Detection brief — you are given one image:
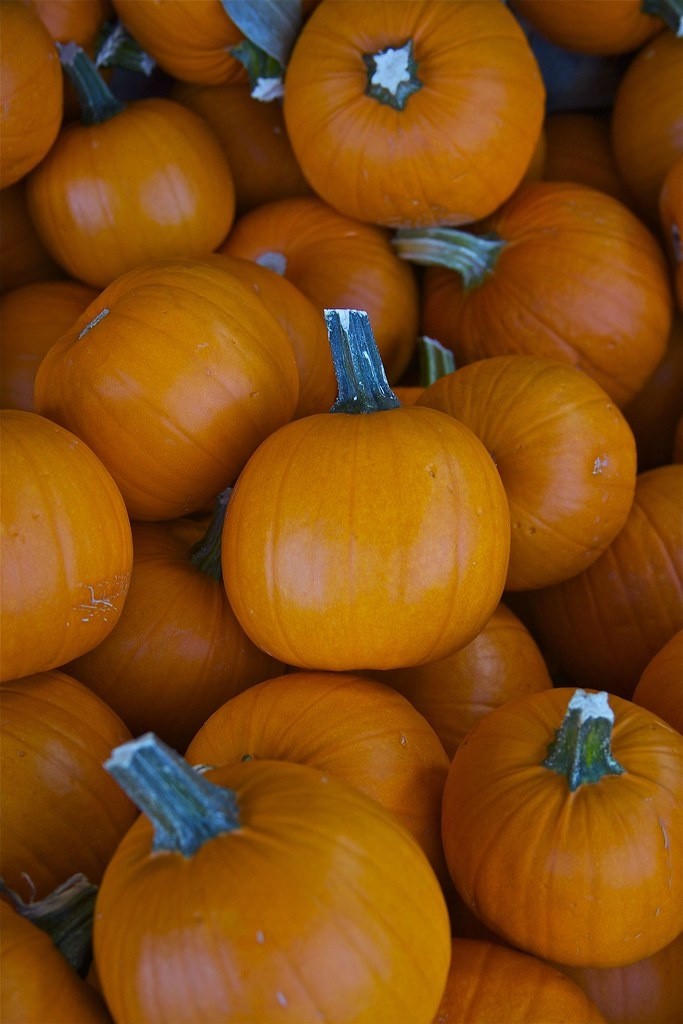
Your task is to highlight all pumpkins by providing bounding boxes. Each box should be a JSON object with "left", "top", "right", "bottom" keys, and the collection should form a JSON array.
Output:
[{"left": 2, "top": 0, "right": 683, "bottom": 1023}]
